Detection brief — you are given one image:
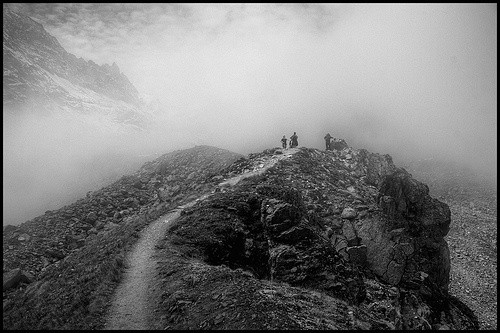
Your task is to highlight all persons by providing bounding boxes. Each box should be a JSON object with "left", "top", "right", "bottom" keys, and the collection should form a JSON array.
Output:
[
  {"left": 291, "top": 132, "right": 298, "bottom": 146},
  {"left": 323, "top": 133, "right": 333, "bottom": 148},
  {"left": 280, "top": 136, "right": 287, "bottom": 147}
]
